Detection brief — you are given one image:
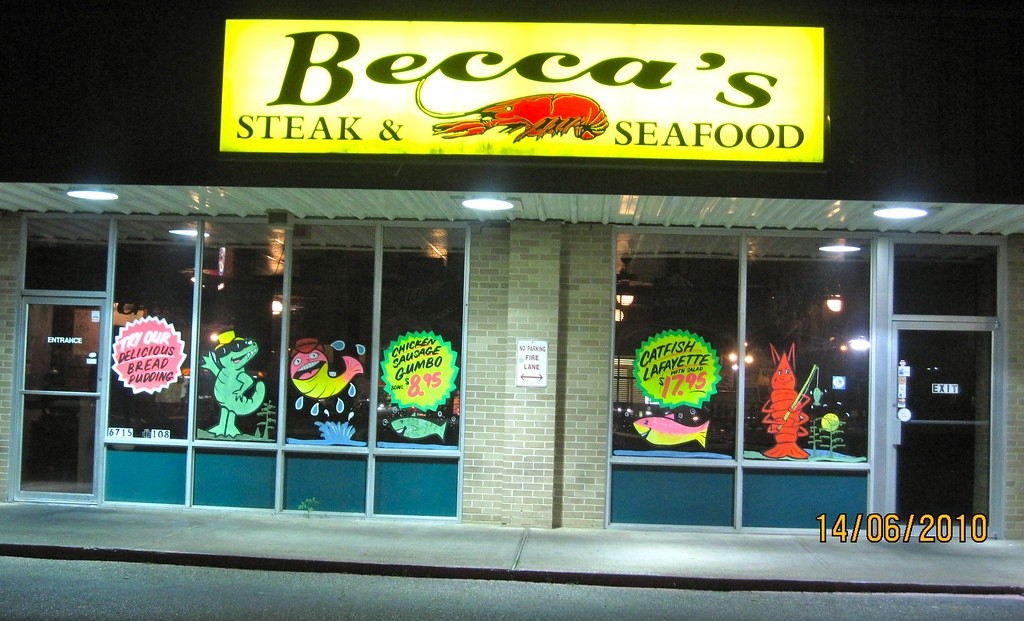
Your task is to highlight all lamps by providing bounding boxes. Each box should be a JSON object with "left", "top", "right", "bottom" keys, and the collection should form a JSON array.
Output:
[
  {"left": 825, "top": 286, "right": 843, "bottom": 312},
  {"left": 615, "top": 309, "right": 624, "bottom": 323},
  {"left": 616, "top": 281, "right": 634, "bottom": 306},
  {"left": 270, "top": 300, "right": 283, "bottom": 316}
]
